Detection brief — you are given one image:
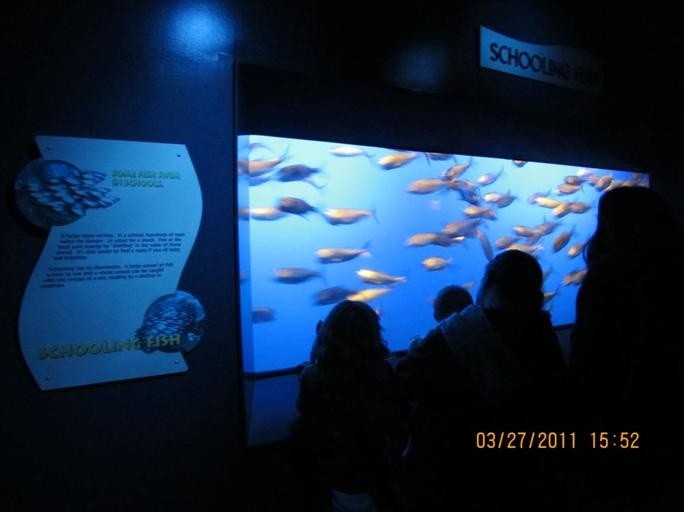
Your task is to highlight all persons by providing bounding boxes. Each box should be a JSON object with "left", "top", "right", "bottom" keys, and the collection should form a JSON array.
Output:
[{"left": 282, "top": 184, "right": 682, "bottom": 512}]
